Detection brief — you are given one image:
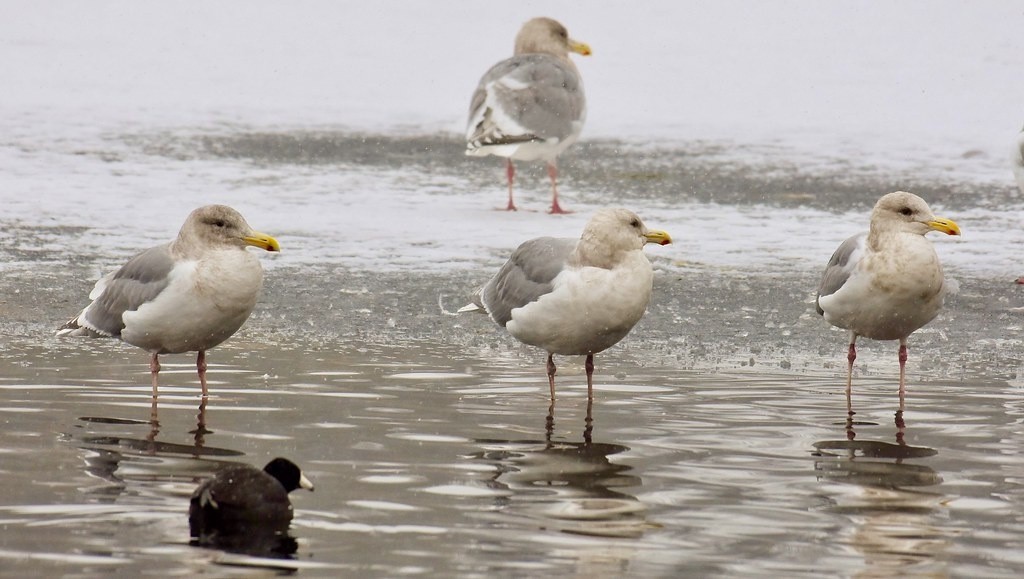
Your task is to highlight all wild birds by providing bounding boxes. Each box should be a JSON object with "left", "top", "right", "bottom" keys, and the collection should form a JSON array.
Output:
[
  {"left": 457, "top": 205, "right": 674, "bottom": 440},
  {"left": 815, "top": 190, "right": 962, "bottom": 428},
  {"left": 464, "top": 16, "right": 592, "bottom": 214},
  {"left": 187, "top": 456, "right": 316, "bottom": 555},
  {"left": 58, "top": 203, "right": 283, "bottom": 397}
]
